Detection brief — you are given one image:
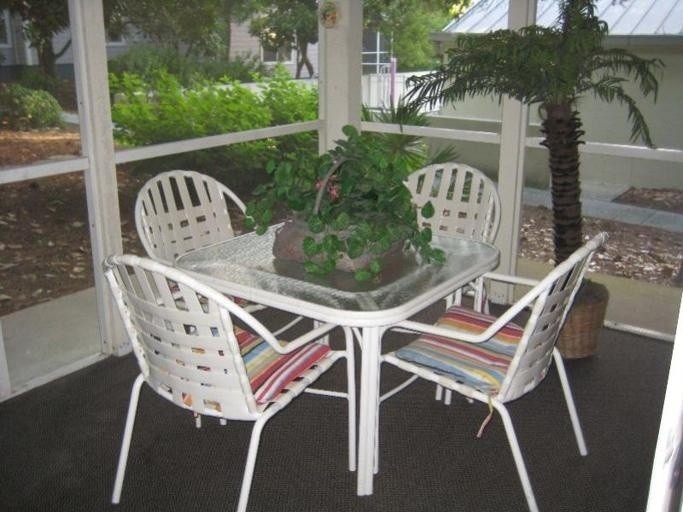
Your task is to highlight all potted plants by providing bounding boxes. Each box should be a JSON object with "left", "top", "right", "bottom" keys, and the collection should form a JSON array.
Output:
[
  {"left": 244, "top": 125, "right": 446, "bottom": 286},
  {"left": 401, "top": 0, "right": 666, "bottom": 360}
]
